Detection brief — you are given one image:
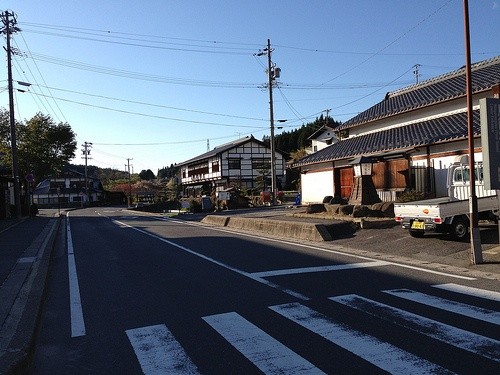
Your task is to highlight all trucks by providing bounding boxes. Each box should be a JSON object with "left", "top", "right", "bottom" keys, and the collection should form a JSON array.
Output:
[{"left": 391, "top": 161, "right": 500, "bottom": 241}]
